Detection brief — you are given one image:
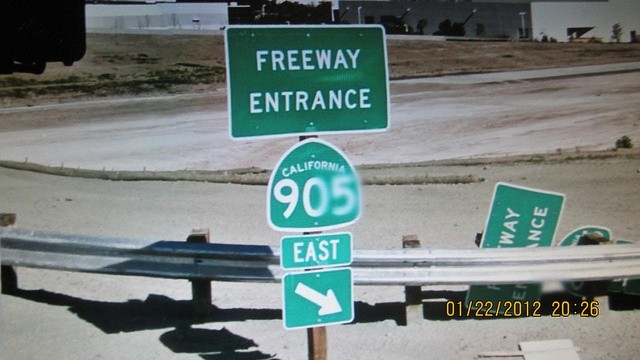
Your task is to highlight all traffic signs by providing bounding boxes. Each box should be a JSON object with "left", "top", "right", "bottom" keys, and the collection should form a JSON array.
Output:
[
  {"left": 280, "top": 233, "right": 354, "bottom": 270},
  {"left": 464, "top": 182, "right": 568, "bottom": 317},
  {"left": 267, "top": 138, "right": 363, "bottom": 232},
  {"left": 229, "top": 25, "right": 390, "bottom": 140},
  {"left": 559, "top": 225, "right": 611, "bottom": 300}
]
